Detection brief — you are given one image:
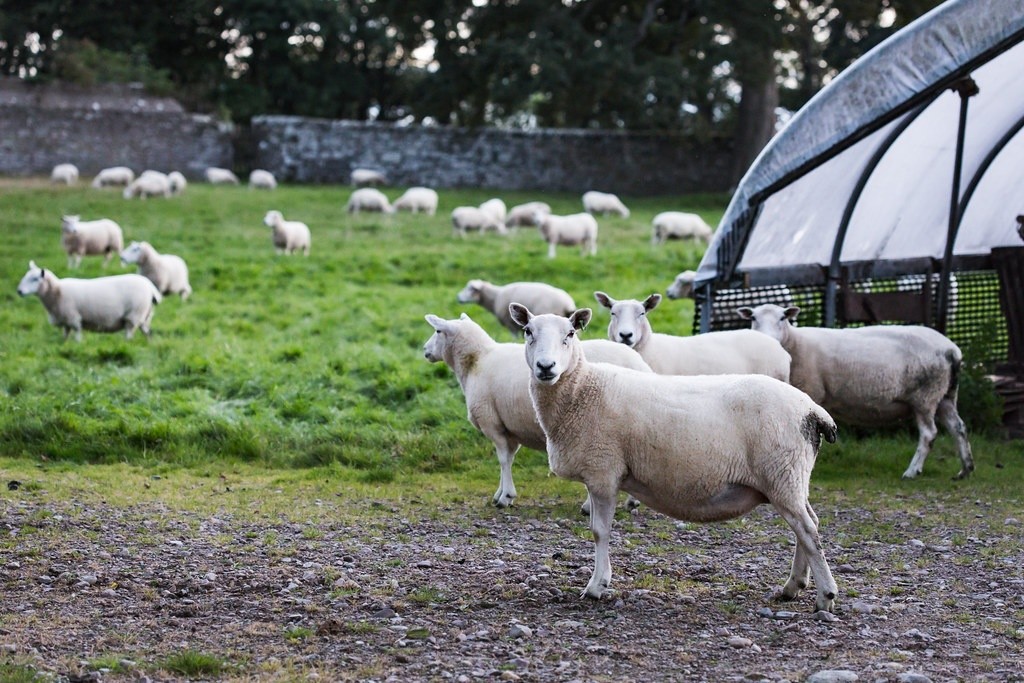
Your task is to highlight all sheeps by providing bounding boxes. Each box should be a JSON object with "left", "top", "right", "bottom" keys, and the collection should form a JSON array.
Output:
[
  {"left": 450, "top": 199, "right": 552, "bottom": 237},
  {"left": 206, "top": 167, "right": 277, "bottom": 188},
  {"left": 582, "top": 191, "right": 630, "bottom": 218},
  {"left": 595, "top": 290, "right": 792, "bottom": 384},
  {"left": 350, "top": 169, "right": 384, "bottom": 189},
  {"left": 61, "top": 214, "right": 126, "bottom": 270},
  {"left": 120, "top": 240, "right": 193, "bottom": 301},
  {"left": 92, "top": 166, "right": 134, "bottom": 188},
  {"left": 509, "top": 303, "right": 838, "bottom": 613},
  {"left": 457, "top": 279, "right": 576, "bottom": 338},
  {"left": 652, "top": 211, "right": 713, "bottom": 246},
  {"left": 263, "top": 210, "right": 311, "bottom": 257},
  {"left": 16, "top": 260, "right": 162, "bottom": 343},
  {"left": 737, "top": 303, "right": 977, "bottom": 481},
  {"left": 533, "top": 210, "right": 599, "bottom": 258},
  {"left": 123, "top": 170, "right": 186, "bottom": 199},
  {"left": 423, "top": 312, "right": 653, "bottom": 509},
  {"left": 52, "top": 164, "right": 79, "bottom": 185},
  {"left": 347, "top": 188, "right": 392, "bottom": 213},
  {"left": 392, "top": 186, "right": 439, "bottom": 216}
]
